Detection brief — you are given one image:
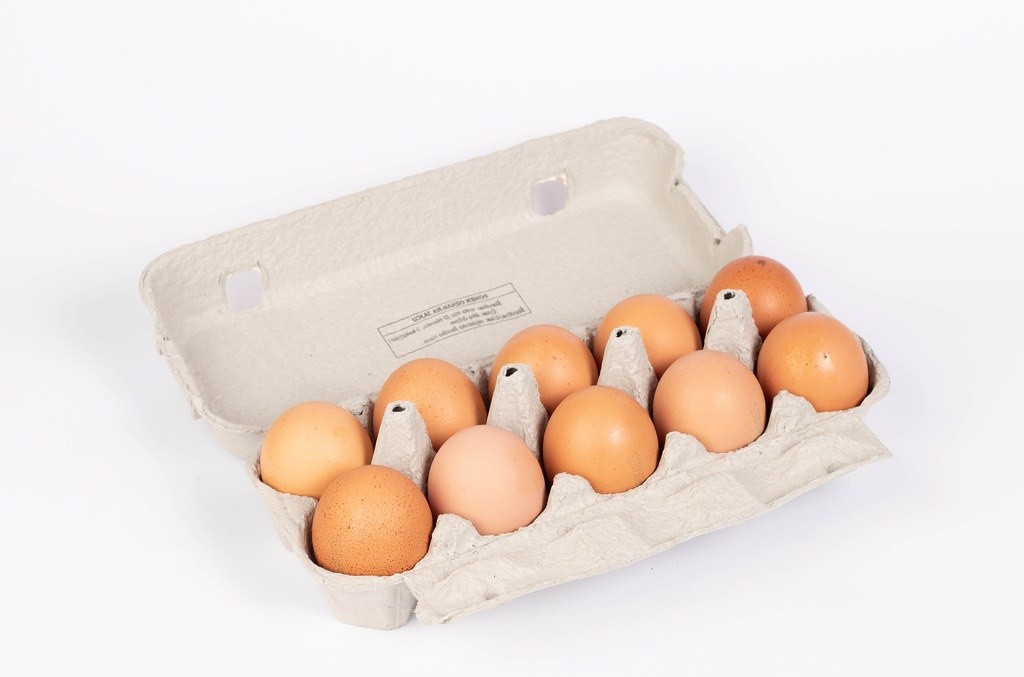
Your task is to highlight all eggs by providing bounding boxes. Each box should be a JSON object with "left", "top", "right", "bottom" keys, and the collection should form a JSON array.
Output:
[{"left": 256, "top": 255, "right": 873, "bottom": 578}]
